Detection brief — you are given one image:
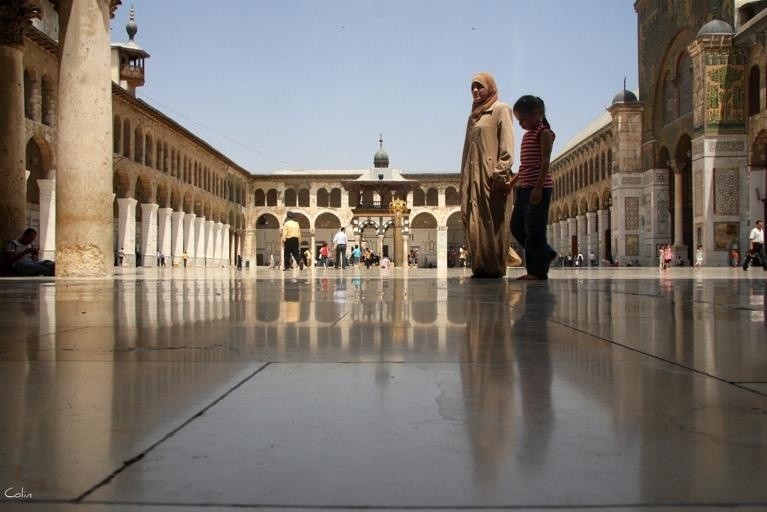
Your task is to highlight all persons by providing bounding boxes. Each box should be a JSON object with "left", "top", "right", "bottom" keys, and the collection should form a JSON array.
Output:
[
  {"left": 157, "top": 249, "right": 165, "bottom": 267},
  {"left": 183, "top": 251, "right": 189, "bottom": 268},
  {"left": 742, "top": 219, "right": 767, "bottom": 271},
  {"left": 613, "top": 254, "right": 640, "bottom": 266},
  {"left": 449, "top": 245, "right": 471, "bottom": 268},
  {"left": 695, "top": 244, "right": 704, "bottom": 269},
  {"left": 731, "top": 248, "right": 740, "bottom": 266},
  {"left": 268, "top": 254, "right": 275, "bottom": 270},
  {"left": 459, "top": 72, "right": 558, "bottom": 280},
  {"left": 281, "top": 211, "right": 420, "bottom": 271},
  {"left": 5, "top": 228, "right": 55, "bottom": 277},
  {"left": 118, "top": 247, "right": 125, "bottom": 266},
  {"left": 458, "top": 280, "right": 554, "bottom": 494},
  {"left": 658, "top": 243, "right": 673, "bottom": 271},
  {"left": 319, "top": 278, "right": 396, "bottom": 321},
  {"left": 558, "top": 251, "right": 597, "bottom": 267}
]
